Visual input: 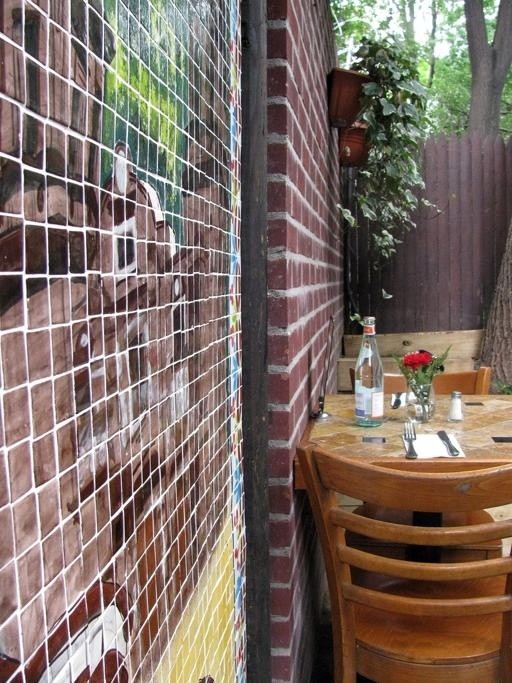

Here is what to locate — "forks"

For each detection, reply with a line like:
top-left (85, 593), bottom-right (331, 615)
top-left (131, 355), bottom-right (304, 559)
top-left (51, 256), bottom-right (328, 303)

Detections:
top-left (403, 421), bottom-right (418, 460)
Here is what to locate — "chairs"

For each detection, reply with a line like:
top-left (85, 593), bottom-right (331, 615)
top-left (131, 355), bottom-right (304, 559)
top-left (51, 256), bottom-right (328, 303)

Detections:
top-left (342, 366), bottom-right (504, 551)
top-left (295, 440), bottom-right (512, 683)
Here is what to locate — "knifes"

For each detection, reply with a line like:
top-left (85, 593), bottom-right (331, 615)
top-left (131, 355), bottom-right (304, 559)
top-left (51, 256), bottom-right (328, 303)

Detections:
top-left (437, 429), bottom-right (460, 457)
top-left (391, 390), bottom-right (402, 410)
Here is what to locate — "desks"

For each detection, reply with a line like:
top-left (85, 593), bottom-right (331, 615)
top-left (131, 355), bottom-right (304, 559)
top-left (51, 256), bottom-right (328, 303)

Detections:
top-left (296, 393), bottom-right (511, 564)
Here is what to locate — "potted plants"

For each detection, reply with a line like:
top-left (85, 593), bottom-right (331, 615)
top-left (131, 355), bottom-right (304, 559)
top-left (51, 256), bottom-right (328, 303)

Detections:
top-left (328, 31), bottom-right (441, 329)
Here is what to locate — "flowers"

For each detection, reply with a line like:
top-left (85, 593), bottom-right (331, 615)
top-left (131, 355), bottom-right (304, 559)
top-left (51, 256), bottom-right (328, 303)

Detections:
top-left (391, 345), bottom-right (456, 412)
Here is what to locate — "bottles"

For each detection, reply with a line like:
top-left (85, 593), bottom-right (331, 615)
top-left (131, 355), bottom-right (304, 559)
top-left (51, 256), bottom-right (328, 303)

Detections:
top-left (353, 312), bottom-right (385, 427)
top-left (448, 389), bottom-right (465, 423)
